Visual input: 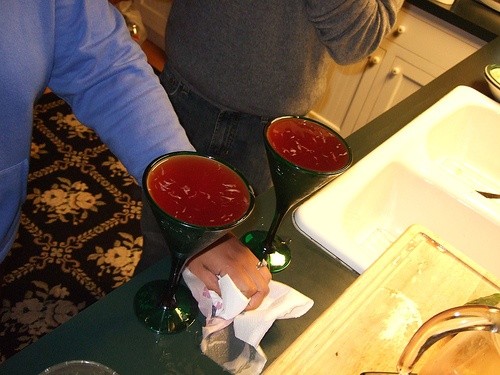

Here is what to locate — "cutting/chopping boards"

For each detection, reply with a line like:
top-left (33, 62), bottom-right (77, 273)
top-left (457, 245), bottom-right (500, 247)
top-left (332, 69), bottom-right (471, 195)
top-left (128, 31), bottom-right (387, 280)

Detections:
top-left (261, 223), bottom-right (500, 375)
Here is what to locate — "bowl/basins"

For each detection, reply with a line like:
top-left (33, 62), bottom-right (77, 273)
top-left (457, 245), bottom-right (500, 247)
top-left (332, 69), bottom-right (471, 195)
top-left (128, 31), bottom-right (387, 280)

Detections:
top-left (483, 63), bottom-right (500, 102)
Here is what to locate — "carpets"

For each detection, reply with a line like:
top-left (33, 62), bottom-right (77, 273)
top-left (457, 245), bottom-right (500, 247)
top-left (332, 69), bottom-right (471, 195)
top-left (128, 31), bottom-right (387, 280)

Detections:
top-left (0, 63), bottom-right (162, 361)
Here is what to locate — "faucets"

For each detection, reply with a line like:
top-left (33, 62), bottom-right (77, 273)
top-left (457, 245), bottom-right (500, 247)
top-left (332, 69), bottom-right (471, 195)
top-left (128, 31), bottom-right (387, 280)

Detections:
top-left (396, 306), bottom-right (500, 375)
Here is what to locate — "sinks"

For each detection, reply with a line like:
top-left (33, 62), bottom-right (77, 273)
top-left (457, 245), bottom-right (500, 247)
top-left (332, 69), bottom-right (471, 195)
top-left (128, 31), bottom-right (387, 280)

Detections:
top-left (335, 161), bottom-right (500, 273)
top-left (415, 101), bottom-right (499, 210)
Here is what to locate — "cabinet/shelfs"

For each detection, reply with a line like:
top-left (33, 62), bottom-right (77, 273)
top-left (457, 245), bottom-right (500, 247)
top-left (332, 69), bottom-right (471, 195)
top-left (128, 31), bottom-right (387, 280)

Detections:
top-left (306, 1), bottom-right (487, 136)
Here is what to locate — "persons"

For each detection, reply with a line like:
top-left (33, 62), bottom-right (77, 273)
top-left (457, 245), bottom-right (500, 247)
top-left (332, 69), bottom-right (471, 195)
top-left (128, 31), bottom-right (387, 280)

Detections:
top-left (131, 0), bottom-right (404, 278)
top-left (0, 0), bottom-right (272, 312)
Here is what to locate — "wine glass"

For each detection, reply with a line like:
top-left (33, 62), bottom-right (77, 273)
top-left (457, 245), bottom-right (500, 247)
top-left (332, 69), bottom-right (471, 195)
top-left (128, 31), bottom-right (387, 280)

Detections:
top-left (240, 114), bottom-right (353, 273)
top-left (134, 150), bottom-right (256, 335)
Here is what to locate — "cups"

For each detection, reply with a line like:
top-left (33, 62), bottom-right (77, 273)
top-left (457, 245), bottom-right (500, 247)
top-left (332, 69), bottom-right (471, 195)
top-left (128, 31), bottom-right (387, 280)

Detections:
top-left (398, 305), bottom-right (500, 375)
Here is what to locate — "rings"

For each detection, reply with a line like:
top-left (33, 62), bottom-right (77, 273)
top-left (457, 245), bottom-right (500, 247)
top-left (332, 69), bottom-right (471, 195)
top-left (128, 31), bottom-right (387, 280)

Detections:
top-left (256, 259), bottom-right (267, 270)
